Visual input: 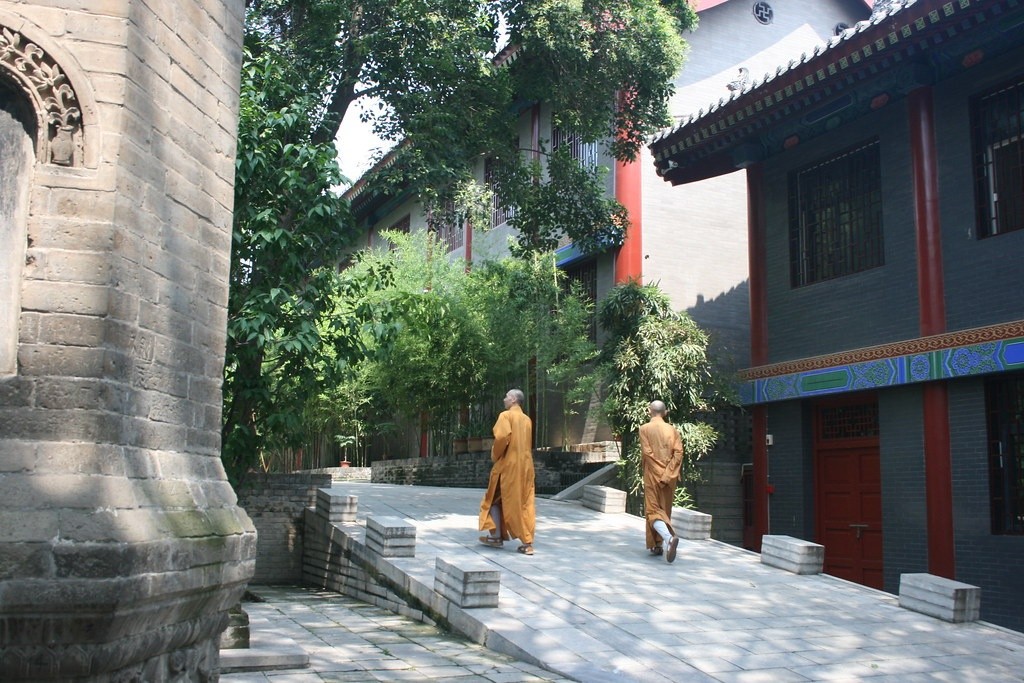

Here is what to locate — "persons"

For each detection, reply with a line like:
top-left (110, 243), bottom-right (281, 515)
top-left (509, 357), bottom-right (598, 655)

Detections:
top-left (478, 388), bottom-right (536, 556)
top-left (639, 399), bottom-right (684, 564)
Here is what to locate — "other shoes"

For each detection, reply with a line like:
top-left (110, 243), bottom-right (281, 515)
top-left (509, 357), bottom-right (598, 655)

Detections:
top-left (650, 546), bottom-right (664, 556)
top-left (478, 535), bottom-right (503, 546)
top-left (516, 544), bottom-right (534, 556)
top-left (666, 536), bottom-right (680, 565)
